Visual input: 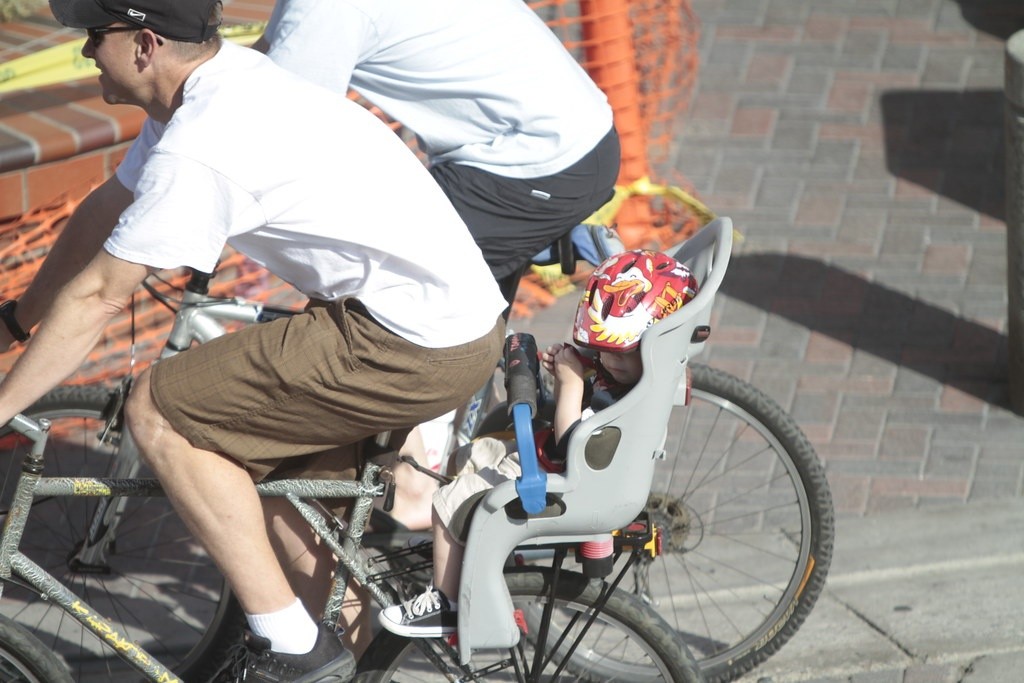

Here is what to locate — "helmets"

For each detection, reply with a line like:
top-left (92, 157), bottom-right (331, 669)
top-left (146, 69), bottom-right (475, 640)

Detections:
top-left (573, 249), bottom-right (701, 352)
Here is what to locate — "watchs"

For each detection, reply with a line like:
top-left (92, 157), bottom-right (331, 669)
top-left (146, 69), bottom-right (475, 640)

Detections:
top-left (0, 300), bottom-right (30, 342)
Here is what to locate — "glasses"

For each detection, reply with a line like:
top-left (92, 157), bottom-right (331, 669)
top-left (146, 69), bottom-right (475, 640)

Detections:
top-left (88, 25), bottom-right (164, 47)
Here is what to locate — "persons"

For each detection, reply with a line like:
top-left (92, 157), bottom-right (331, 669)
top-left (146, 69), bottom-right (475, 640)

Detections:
top-left (377, 249), bottom-right (699, 637)
top-left (250, 0), bottom-right (621, 549)
top-left (0, 0), bottom-right (509, 682)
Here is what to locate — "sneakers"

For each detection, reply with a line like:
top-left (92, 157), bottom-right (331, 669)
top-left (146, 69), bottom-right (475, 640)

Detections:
top-left (402, 536), bottom-right (436, 575)
top-left (207, 622), bottom-right (356, 683)
top-left (376, 578), bottom-right (458, 639)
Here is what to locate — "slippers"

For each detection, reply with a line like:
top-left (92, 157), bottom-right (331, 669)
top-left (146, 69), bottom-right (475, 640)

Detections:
top-left (330, 506), bottom-right (437, 571)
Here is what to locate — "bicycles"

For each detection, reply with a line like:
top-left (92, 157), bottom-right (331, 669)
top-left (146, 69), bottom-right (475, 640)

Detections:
top-left (0, 190), bottom-right (837, 683)
top-left (1, 415), bottom-right (705, 683)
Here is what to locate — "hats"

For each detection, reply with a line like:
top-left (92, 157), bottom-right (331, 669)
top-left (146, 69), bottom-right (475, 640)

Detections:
top-left (47, 0), bottom-right (223, 44)
